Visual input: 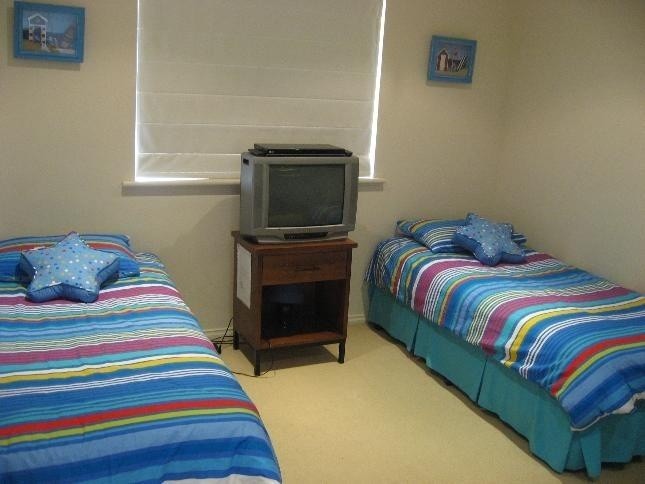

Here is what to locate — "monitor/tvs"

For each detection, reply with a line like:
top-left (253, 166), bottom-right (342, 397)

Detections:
top-left (239, 152), bottom-right (359, 245)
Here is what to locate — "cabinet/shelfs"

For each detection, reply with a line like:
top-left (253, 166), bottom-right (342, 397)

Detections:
top-left (229, 230), bottom-right (358, 376)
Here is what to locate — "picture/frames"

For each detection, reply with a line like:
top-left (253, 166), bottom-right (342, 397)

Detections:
top-left (13, 0), bottom-right (86, 63)
top-left (427, 34), bottom-right (477, 84)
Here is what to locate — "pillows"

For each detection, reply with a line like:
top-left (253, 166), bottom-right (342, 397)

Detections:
top-left (396, 212), bottom-right (527, 266)
top-left (0, 232), bottom-right (142, 304)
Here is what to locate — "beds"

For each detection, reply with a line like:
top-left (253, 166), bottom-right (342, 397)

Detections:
top-left (0, 233), bottom-right (281, 483)
top-left (369, 215), bottom-right (645, 480)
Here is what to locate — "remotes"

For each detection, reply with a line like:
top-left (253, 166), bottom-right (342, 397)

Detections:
top-left (248, 148), bottom-right (266, 157)
